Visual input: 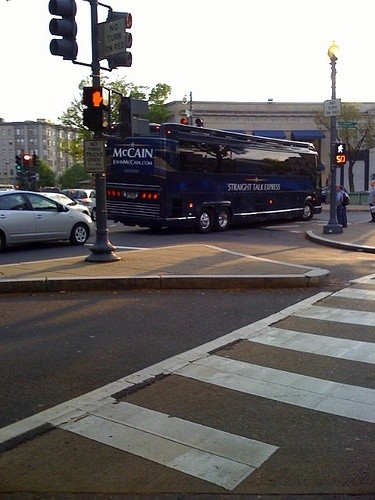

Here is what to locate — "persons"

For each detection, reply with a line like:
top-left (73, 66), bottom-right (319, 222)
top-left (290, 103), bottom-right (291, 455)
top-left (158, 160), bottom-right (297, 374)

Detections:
top-left (70, 191), bottom-right (96, 199)
top-left (336, 185), bottom-right (349, 228)
top-left (369, 179), bottom-right (375, 222)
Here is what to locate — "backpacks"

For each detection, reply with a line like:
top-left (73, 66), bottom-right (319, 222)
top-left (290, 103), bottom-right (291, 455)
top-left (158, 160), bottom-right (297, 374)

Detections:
top-left (339, 191), bottom-right (350, 206)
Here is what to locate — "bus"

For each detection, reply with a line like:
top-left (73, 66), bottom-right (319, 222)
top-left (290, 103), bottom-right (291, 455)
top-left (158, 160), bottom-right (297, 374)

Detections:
top-left (95, 122), bottom-right (325, 234)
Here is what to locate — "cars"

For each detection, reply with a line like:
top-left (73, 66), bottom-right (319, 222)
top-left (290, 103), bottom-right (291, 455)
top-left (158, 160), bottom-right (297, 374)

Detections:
top-left (0, 190), bottom-right (97, 254)
top-left (0, 184), bottom-right (96, 221)
top-left (321, 184), bottom-right (350, 207)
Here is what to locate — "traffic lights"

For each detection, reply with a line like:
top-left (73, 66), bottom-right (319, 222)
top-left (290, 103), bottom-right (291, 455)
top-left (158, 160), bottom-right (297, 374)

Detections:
top-left (100, 86), bottom-right (110, 111)
top-left (88, 87), bottom-right (102, 109)
top-left (15, 154), bottom-right (23, 170)
top-left (334, 141), bottom-right (347, 166)
top-left (33, 154), bottom-right (40, 166)
top-left (48, 0), bottom-right (78, 60)
top-left (106, 8), bottom-right (132, 69)
top-left (94, 108), bottom-right (111, 131)
top-left (180, 117), bottom-right (189, 125)
top-left (22, 153), bottom-right (30, 163)
top-left (195, 118), bottom-right (204, 128)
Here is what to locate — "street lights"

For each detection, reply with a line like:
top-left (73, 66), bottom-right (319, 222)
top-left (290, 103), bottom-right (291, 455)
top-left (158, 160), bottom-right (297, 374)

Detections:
top-left (322, 40), bottom-right (344, 235)
top-left (182, 90), bottom-right (193, 126)
